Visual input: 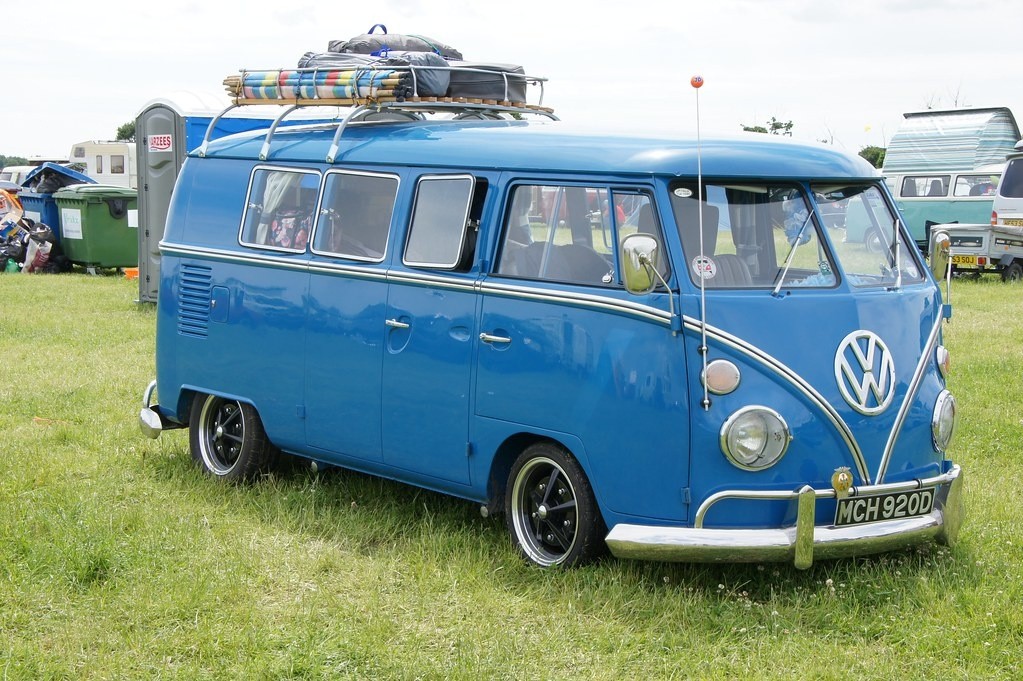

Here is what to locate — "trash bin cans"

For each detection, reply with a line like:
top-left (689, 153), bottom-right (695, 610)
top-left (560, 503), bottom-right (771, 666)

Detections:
top-left (52, 185), bottom-right (138, 276)
top-left (17, 162), bottom-right (99, 247)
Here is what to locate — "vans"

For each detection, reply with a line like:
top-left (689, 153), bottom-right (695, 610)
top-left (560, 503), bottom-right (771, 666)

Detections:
top-left (1, 166), bottom-right (41, 192)
top-left (135, 63), bottom-right (965, 576)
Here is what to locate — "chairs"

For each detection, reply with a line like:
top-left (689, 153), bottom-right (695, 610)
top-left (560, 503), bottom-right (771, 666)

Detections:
top-left (525, 240), bottom-right (613, 283)
top-left (927, 180), bottom-right (942, 196)
top-left (903, 179), bottom-right (917, 197)
top-left (693, 254), bottom-right (755, 287)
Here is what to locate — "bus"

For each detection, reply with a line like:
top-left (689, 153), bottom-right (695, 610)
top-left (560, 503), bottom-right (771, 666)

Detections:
top-left (844, 164), bottom-right (1006, 257)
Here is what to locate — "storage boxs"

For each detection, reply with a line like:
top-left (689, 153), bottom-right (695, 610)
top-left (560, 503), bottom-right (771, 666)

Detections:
top-left (0, 207), bottom-right (31, 243)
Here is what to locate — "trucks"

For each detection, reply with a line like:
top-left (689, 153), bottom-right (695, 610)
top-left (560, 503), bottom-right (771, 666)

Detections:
top-left (929, 153), bottom-right (1022, 283)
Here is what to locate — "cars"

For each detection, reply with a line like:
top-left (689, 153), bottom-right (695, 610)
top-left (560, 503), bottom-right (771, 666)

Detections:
top-left (815, 195), bottom-right (846, 226)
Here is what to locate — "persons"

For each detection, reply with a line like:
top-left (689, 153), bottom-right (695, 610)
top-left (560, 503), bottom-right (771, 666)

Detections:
top-left (602, 199), bottom-right (626, 227)
